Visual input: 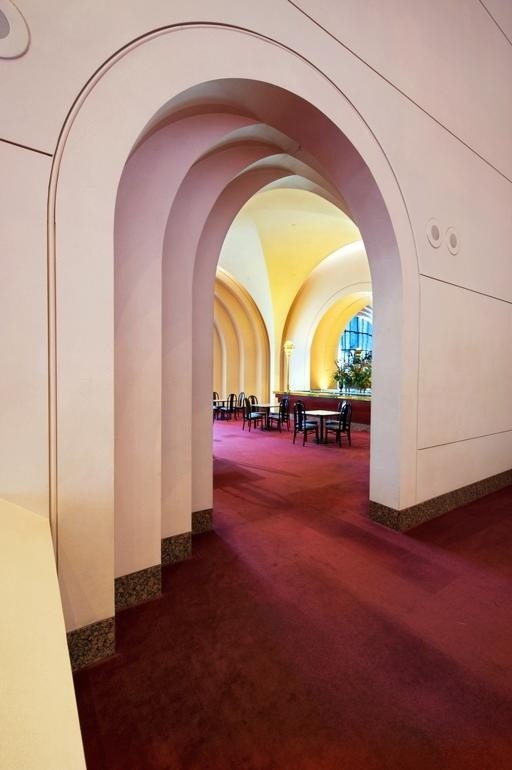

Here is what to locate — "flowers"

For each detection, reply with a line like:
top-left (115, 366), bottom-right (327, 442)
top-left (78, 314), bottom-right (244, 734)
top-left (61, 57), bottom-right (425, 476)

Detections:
top-left (332, 350), bottom-right (372, 390)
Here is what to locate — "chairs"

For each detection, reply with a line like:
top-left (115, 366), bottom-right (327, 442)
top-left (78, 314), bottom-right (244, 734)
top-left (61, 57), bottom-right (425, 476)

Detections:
top-left (212, 392), bottom-right (354, 449)
